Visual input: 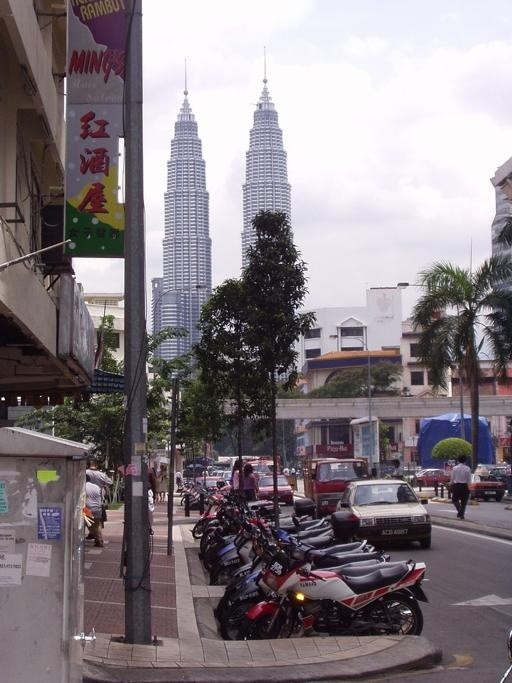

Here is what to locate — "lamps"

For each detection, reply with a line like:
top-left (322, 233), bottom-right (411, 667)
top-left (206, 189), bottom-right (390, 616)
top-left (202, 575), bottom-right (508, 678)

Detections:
top-left (0, 238), bottom-right (76, 272)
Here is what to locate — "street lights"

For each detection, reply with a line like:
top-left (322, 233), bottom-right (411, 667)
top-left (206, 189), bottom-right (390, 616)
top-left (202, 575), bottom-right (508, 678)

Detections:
top-left (397, 283), bottom-right (465, 441)
top-left (154, 286), bottom-right (206, 380)
top-left (329, 334), bottom-right (373, 480)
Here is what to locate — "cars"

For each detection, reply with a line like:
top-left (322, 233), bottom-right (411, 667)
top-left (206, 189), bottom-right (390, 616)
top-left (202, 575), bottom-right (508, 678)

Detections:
top-left (466, 467), bottom-right (512, 503)
top-left (333, 480), bottom-right (431, 551)
top-left (195, 459), bottom-right (280, 492)
top-left (256, 475), bottom-right (294, 506)
top-left (414, 468), bottom-right (450, 486)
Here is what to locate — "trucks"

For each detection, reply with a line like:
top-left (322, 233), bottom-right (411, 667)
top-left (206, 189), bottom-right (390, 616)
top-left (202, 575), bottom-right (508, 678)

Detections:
top-left (300, 456), bottom-right (370, 519)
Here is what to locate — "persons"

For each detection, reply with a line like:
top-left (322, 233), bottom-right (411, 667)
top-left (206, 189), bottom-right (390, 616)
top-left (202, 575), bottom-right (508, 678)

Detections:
top-left (244, 463), bottom-right (259, 501)
top-left (231, 459), bottom-right (240, 496)
top-left (86, 458), bottom-right (113, 487)
top-left (176, 470), bottom-right (182, 489)
top-left (449, 454), bottom-right (471, 520)
top-left (86, 474), bottom-right (104, 547)
top-left (147, 464), bottom-right (167, 534)
top-left (392, 459), bottom-right (403, 480)
top-left (371, 463), bottom-right (378, 479)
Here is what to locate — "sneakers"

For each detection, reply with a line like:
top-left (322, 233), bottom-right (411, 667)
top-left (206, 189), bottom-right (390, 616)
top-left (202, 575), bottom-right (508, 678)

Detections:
top-left (86, 534), bottom-right (103, 547)
top-left (457, 513), bottom-right (464, 519)
